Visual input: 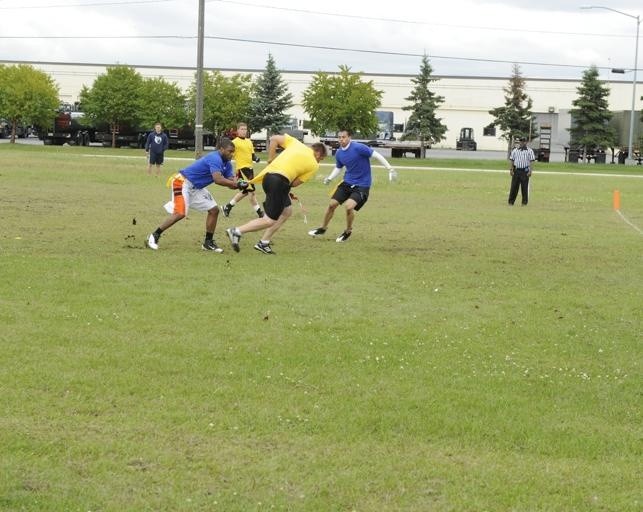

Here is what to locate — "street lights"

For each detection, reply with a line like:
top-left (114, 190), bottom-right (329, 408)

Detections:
top-left (577, 3), bottom-right (643, 166)
top-left (612, 66), bottom-right (643, 76)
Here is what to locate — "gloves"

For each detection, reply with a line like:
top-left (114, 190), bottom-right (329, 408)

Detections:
top-left (236, 179), bottom-right (249, 191)
top-left (322, 177), bottom-right (332, 185)
top-left (387, 168), bottom-right (398, 183)
top-left (255, 156), bottom-right (261, 163)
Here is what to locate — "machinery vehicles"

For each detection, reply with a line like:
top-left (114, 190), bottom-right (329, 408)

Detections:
top-left (0, 101), bottom-right (212, 149)
top-left (456, 126), bottom-right (479, 151)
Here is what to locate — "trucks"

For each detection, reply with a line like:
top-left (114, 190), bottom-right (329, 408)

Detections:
top-left (246, 111), bottom-right (300, 154)
top-left (511, 104), bottom-right (643, 167)
top-left (369, 108), bottom-right (394, 146)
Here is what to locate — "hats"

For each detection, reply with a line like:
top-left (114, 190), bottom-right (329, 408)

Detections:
top-left (518, 136), bottom-right (528, 142)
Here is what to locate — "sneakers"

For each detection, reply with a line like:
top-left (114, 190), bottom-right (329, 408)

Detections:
top-left (200, 238), bottom-right (225, 254)
top-left (220, 201), bottom-right (232, 218)
top-left (224, 226), bottom-right (242, 253)
top-left (334, 229), bottom-right (352, 243)
top-left (145, 231), bottom-right (160, 250)
top-left (305, 225), bottom-right (327, 239)
top-left (253, 239), bottom-right (277, 255)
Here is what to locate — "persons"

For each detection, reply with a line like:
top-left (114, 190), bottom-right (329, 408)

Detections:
top-left (145, 123), bottom-right (169, 176)
top-left (509, 137), bottom-right (535, 205)
top-left (84, 131), bottom-right (90, 146)
top-left (144, 132), bottom-right (148, 148)
top-left (307, 127), bottom-right (397, 243)
top-left (138, 132), bottom-right (143, 148)
top-left (220, 123), bottom-right (264, 218)
top-left (78, 131), bottom-right (83, 146)
top-left (145, 139), bottom-right (249, 253)
top-left (225, 134), bottom-right (328, 254)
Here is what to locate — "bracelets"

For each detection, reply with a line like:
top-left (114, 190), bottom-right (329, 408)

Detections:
top-left (510, 169), bottom-right (514, 171)
